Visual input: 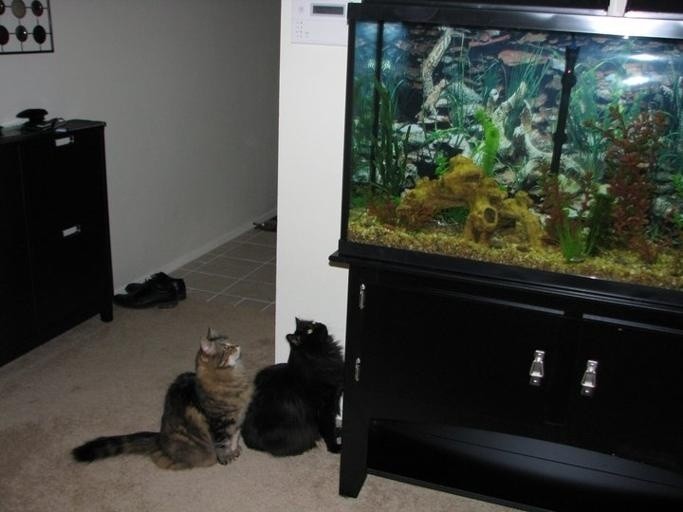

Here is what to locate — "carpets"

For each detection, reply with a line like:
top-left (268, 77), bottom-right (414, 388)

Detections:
top-left (0, 296), bottom-right (532, 511)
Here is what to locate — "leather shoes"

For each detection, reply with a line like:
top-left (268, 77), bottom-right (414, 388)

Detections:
top-left (113, 277), bottom-right (177, 308)
top-left (125, 271), bottom-right (186, 301)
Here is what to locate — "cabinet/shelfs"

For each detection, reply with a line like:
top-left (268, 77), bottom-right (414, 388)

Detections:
top-left (328, 249), bottom-right (683, 511)
top-left (0, 119), bottom-right (114, 368)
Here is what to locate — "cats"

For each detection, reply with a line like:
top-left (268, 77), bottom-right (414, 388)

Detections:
top-left (241, 315), bottom-right (347, 458)
top-left (69, 328), bottom-right (246, 470)
